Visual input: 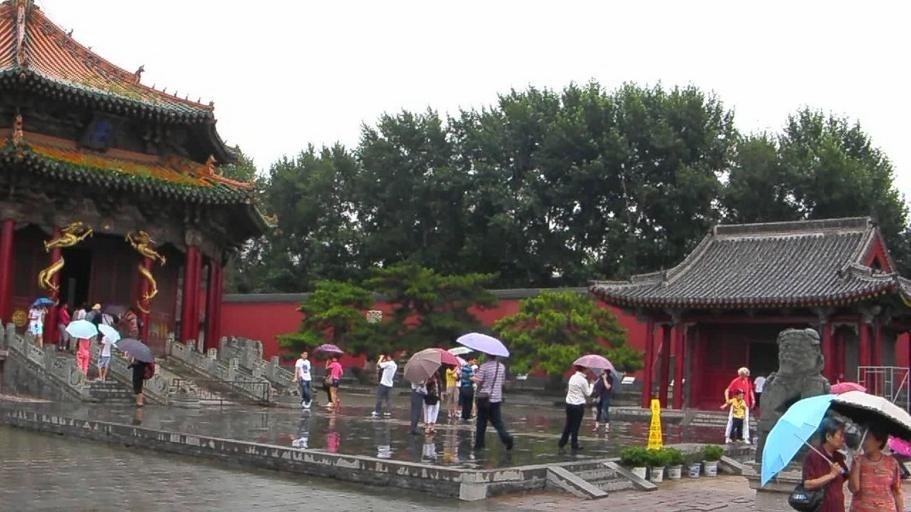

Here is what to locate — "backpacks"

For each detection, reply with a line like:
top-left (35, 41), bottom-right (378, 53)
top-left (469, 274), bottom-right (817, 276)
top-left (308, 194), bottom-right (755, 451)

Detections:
top-left (144, 361), bottom-right (154, 380)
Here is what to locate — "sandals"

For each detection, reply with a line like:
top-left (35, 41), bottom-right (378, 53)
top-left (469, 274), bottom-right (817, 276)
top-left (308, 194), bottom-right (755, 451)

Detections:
top-left (592, 426), bottom-right (610, 433)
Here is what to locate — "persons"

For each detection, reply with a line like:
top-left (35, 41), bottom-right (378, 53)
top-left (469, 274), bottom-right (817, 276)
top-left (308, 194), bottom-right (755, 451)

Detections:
top-left (293, 350), bottom-right (315, 410)
top-left (371, 351), bottom-right (399, 415)
top-left (800, 416), bottom-right (852, 512)
top-left (13, 302), bottom-right (139, 382)
top-left (847, 424), bottom-right (905, 511)
top-left (721, 389), bottom-right (748, 441)
top-left (326, 355), bottom-right (346, 408)
top-left (752, 370), bottom-right (767, 406)
top-left (125, 357), bottom-right (147, 407)
top-left (591, 369), bottom-right (614, 433)
top-left (472, 354), bottom-right (516, 450)
top-left (555, 366), bottom-right (595, 450)
top-left (404, 356), bottom-right (479, 437)
top-left (725, 366), bottom-right (757, 444)
top-left (377, 352), bottom-right (387, 383)
top-left (323, 356), bottom-right (334, 407)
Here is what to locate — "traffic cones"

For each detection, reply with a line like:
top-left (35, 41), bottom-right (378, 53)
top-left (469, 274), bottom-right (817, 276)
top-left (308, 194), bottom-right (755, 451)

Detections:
top-left (647, 398), bottom-right (667, 452)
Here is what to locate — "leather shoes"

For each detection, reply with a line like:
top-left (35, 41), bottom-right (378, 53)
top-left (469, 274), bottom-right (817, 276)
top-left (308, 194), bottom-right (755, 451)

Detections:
top-left (472, 441), bottom-right (486, 451)
top-left (557, 444), bottom-right (583, 450)
top-left (504, 435), bottom-right (514, 451)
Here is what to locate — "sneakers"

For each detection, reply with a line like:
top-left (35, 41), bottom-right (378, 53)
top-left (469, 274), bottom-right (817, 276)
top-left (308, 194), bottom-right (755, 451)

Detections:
top-left (408, 427), bottom-right (438, 435)
top-left (300, 399), bottom-right (313, 409)
top-left (371, 411), bottom-right (392, 417)
top-left (447, 409), bottom-right (476, 421)
top-left (724, 436), bottom-right (752, 446)
top-left (324, 400), bottom-right (341, 410)
top-left (94, 377), bottom-right (106, 384)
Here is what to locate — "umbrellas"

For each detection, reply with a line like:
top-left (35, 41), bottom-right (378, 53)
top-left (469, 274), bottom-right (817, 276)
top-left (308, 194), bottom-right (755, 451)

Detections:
top-left (572, 353), bottom-right (619, 372)
top-left (447, 345), bottom-right (475, 356)
top-left (593, 368), bottom-right (622, 391)
top-left (829, 390), bottom-right (911, 457)
top-left (312, 343), bottom-right (346, 356)
top-left (31, 298), bottom-right (55, 312)
top-left (403, 347), bottom-right (441, 383)
top-left (830, 379), bottom-right (869, 394)
top-left (456, 331), bottom-right (512, 360)
top-left (115, 338), bottom-right (155, 364)
top-left (433, 347), bottom-right (462, 366)
top-left (759, 394), bottom-right (837, 486)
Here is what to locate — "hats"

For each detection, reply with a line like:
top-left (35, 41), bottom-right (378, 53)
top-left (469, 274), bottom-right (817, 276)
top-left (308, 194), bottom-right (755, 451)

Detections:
top-left (91, 302), bottom-right (102, 310)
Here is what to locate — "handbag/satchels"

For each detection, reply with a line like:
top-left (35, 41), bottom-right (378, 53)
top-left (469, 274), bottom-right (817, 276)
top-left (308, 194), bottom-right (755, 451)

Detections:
top-left (425, 394), bottom-right (437, 405)
top-left (788, 483), bottom-right (825, 512)
top-left (474, 392), bottom-right (490, 404)
top-left (324, 376), bottom-right (333, 384)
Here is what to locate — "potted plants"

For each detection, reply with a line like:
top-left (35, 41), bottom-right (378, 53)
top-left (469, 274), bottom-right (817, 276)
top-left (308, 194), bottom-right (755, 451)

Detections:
top-left (626, 442), bottom-right (648, 484)
top-left (685, 455), bottom-right (704, 481)
top-left (704, 444), bottom-right (725, 479)
top-left (665, 449), bottom-right (684, 481)
top-left (643, 444), bottom-right (667, 485)
